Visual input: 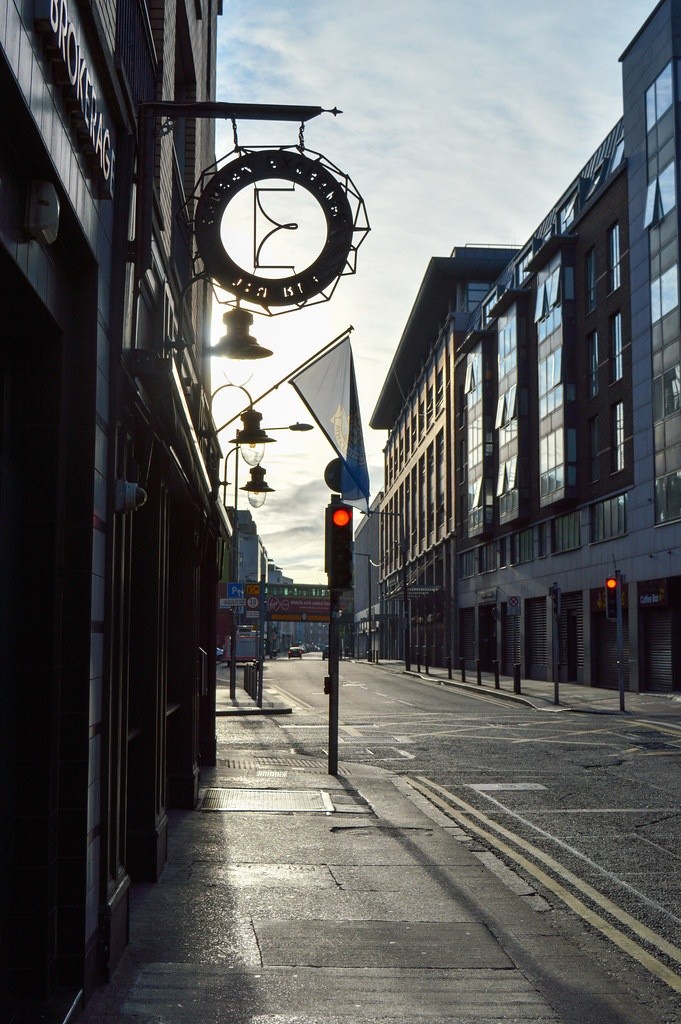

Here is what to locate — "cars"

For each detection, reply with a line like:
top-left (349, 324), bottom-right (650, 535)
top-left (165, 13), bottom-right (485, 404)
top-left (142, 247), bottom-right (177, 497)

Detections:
top-left (321, 645), bottom-right (331, 660)
top-left (287, 647), bottom-right (302, 659)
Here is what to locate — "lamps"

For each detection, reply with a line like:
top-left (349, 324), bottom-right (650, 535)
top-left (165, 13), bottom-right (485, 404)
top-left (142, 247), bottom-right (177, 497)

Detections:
top-left (22, 175), bottom-right (62, 244)
top-left (213, 446), bottom-right (277, 508)
top-left (199, 383), bottom-right (278, 465)
top-left (165, 273), bottom-right (275, 387)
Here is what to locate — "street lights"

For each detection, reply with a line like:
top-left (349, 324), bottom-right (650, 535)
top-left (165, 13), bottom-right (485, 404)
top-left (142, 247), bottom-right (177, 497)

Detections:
top-left (229, 423), bottom-right (314, 700)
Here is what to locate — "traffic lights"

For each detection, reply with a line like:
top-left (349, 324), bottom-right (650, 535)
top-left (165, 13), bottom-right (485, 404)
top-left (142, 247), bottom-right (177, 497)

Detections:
top-left (605, 576), bottom-right (619, 621)
top-left (329, 503), bottom-right (353, 592)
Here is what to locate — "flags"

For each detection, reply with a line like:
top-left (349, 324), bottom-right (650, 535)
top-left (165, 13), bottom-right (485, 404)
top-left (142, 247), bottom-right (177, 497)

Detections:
top-left (289, 336), bottom-right (372, 519)
